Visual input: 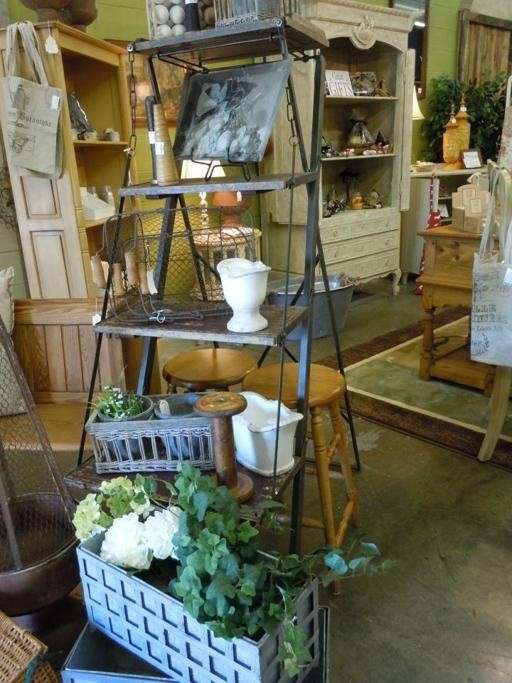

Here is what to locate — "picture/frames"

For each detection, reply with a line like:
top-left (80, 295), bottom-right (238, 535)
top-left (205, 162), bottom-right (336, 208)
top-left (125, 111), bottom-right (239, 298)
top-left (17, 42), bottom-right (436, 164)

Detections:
top-left (101, 37), bottom-right (190, 128)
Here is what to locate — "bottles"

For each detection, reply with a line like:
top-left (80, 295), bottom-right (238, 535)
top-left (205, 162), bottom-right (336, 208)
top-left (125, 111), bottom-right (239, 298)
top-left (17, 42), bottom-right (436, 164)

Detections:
top-left (326, 184), bottom-right (339, 214)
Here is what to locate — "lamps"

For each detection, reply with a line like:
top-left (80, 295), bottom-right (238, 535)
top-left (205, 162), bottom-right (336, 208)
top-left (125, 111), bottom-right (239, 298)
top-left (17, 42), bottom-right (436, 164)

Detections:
top-left (178, 151), bottom-right (227, 242)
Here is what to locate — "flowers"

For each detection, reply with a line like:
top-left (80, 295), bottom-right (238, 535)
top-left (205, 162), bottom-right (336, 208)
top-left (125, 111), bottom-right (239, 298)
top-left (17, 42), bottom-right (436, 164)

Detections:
top-left (89, 384), bottom-right (144, 419)
top-left (71, 473), bottom-right (394, 631)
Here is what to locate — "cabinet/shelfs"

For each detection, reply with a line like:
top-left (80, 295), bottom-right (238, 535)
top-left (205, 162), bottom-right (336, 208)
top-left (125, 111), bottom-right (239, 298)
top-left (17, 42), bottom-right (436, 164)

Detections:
top-left (256, 0), bottom-right (425, 302)
top-left (0, 18), bottom-right (161, 406)
top-left (400, 160), bottom-right (488, 284)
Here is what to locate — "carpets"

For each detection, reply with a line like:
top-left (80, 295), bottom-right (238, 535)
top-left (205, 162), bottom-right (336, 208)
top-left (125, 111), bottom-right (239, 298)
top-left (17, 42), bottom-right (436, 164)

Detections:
top-left (313, 304), bottom-right (511, 472)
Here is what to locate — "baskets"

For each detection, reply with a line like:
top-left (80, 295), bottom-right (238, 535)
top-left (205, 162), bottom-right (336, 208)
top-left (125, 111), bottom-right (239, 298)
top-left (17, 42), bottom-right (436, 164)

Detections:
top-left (0, 611), bottom-right (62, 683)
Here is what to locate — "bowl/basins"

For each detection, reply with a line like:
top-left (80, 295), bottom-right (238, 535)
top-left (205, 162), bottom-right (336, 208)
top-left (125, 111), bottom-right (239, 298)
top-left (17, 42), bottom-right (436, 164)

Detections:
top-left (252, 276), bottom-right (355, 337)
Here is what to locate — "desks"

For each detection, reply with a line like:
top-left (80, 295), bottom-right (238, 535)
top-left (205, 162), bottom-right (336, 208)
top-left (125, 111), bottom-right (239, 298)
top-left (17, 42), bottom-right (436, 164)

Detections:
top-left (192, 227), bottom-right (262, 302)
top-left (412, 226), bottom-right (510, 397)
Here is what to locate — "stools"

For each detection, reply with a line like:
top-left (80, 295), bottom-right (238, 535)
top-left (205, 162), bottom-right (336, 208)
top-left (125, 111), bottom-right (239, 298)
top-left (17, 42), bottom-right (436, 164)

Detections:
top-left (238, 361), bottom-right (359, 555)
top-left (163, 347), bottom-right (259, 396)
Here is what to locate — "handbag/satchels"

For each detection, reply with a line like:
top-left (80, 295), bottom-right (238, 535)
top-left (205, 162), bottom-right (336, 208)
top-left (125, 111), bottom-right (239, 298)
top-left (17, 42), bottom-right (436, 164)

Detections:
top-left (1, 20), bottom-right (66, 181)
top-left (465, 165), bottom-right (512, 368)
